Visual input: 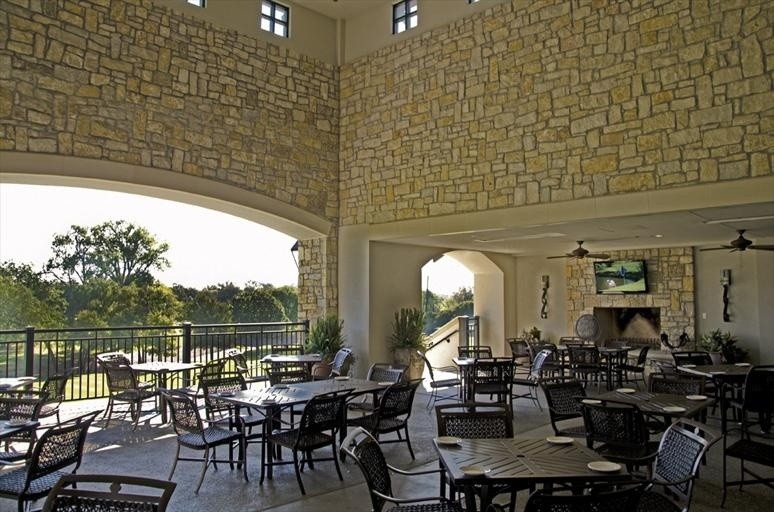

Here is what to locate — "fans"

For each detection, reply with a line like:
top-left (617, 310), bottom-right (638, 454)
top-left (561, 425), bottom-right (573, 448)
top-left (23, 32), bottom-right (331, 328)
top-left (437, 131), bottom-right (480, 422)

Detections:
top-left (546, 240), bottom-right (611, 260)
top-left (699, 229), bottom-right (774, 253)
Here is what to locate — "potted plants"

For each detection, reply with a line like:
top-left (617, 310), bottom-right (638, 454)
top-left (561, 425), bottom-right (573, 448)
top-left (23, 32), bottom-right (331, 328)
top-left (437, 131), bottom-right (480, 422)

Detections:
top-left (385, 306), bottom-right (434, 384)
top-left (697, 330), bottom-right (738, 365)
top-left (303, 316), bottom-right (351, 376)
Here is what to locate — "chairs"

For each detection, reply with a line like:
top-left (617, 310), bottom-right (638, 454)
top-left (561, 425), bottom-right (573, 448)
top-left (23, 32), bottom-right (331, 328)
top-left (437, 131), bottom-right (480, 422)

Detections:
top-left (197, 371), bottom-right (277, 469)
top-left (559, 337), bottom-right (585, 346)
top-left (648, 372), bottom-right (706, 396)
top-left (507, 338), bottom-right (529, 374)
top-left (457, 346), bottom-right (492, 358)
top-left (340, 426), bottom-right (462, 512)
top-left (30, 474), bottom-right (178, 512)
top-left (417, 350), bottom-right (465, 415)
top-left (600, 417), bottom-right (722, 512)
top-left (156, 386), bottom-right (249, 493)
top-left (271, 344), bottom-right (304, 355)
top-left (97, 357), bottom-right (161, 432)
top-left (432, 438), bottom-right (632, 512)
top-left (345, 377), bottom-right (425, 465)
top-left (528, 341), bottom-right (570, 383)
top-left (565, 343), bottom-right (608, 395)
top-left (726, 365), bottom-right (774, 440)
top-left (540, 381), bottom-right (587, 438)
top-left (614, 345), bottom-right (651, 390)
top-left (310, 348), bottom-right (351, 381)
top-left (468, 356), bottom-right (515, 419)
top-left (671, 351), bottom-right (713, 366)
top-left (0, 390), bottom-right (50, 468)
top-left (524, 480), bottom-right (648, 512)
top-left (602, 340), bottom-right (628, 384)
top-left (228, 352), bottom-right (270, 388)
top-left (650, 359), bottom-right (678, 371)
top-left (264, 363), bottom-right (312, 386)
top-left (97, 352), bottom-right (155, 418)
top-left (0, 409), bottom-right (105, 512)
top-left (435, 402), bottom-right (536, 512)
top-left (259, 388), bottom-right (356, 495)
top-left (334, 363), bottom-right (409, 440)
top-left (504, 349), bottom-right (552, 411)
top-left (570, 395), bottom-right (661, 480)
top-left (0, 370), bottom-right (74, 431)
top-left (168, 356), bottom-right (230, 424)
top-left (721, 417), bottom-right (774, 509)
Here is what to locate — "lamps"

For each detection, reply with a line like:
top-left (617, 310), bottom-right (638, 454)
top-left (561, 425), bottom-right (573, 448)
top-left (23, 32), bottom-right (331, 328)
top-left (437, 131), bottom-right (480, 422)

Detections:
top-left (541, 275), bottom-right (549, 321)
top-left (720, 268), bottom-right (732, 322)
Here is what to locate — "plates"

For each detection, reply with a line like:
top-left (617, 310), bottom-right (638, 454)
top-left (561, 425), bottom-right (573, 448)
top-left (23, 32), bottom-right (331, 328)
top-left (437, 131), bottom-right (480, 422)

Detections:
top-left (334, 376), bottom-right (350, 380)
top-left (546, 437), bottom-right (574, 444)
top-left (709, 372), bottom-right (726, 375)
top-left (607, 349), bottom-right (617, 351)
top-left (262, 399), bottom-right (276, 404)
top-left (581, 399), bottom-right (601, 404)
top-left (621, 347), bottom-right (631, 349)
top-left (273, 384), bottom-right (287, 388)
top-left (3, 421), bottom-right (32, 427)
top-left (217, 393), bottom-right (235, 396)
top-left (662, 407), bottom-right (686, 413)
top-left (735, 363), bottom-right (750, 366)
top-left (433, 437), bottom-right (461, 444)
top-left (377, 382), bottom-right (395, 386)
top-left (265, 359), bottom-right (271, 361)
top-left (685, 395), bottom-right (707, 400)
top-left (616, 388), bottom-right (635, 393)
top-left (682, 365), bottom-right (696, 368)
top-left (458, 466), bottom-right (490, 476)
top-left (587, 462), bottom-right (621, 472)
top-left (271, 354), bottom-right (278, 356)
